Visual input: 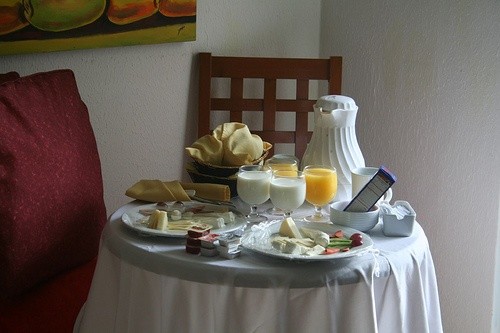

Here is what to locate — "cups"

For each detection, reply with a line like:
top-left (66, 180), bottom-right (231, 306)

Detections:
top-left (271, 154), bottom-right (299, 165)
top-left (351, 167), bottom-right (393, 207)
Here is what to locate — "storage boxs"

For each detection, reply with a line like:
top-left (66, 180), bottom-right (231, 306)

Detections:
top-left (381, 197), bottom-right (418, 238)
top-left (343, 164), bottom-right (399, 212)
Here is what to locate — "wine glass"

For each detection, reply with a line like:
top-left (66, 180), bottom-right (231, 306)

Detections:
top-left (269, 169), bottom-right (307, 221)
top-left (263, 159), bottom-right (299, 215)
top-left (236, 165), bottom-right (272, 223)
top-left (303, 165), bottom-right (337, 222)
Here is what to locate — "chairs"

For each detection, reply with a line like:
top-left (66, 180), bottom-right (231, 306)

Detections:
top-left (0, 70), bottom-right (97, 333)
top-left (196, 47), bottom-right (344, 168)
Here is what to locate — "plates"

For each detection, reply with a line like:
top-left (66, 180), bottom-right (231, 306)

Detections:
top-left (122, 202), bottom-right (247, 237)
top-left (241, 221), bottom-right (374, 262)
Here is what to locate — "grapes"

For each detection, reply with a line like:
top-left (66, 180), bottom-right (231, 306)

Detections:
top-left (350, 234), bottom-right (364, 245)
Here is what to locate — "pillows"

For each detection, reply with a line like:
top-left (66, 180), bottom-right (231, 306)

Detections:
top-left (0, 68), bottom-right (109, 302)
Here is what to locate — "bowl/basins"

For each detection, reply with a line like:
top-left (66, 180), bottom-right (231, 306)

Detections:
top-left (185, 151), bottom-right (269, 196)
top-left (329, 202), bottom-right (380, 233)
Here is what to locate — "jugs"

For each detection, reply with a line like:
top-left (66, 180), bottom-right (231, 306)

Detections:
top-left (301, 94), bottom-right (365, 203)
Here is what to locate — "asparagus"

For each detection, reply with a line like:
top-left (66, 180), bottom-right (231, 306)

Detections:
top-left (327, 239), bottom-right (352, 248)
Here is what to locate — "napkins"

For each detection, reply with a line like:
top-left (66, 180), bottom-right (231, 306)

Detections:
top-left (125, 174), bottom-right (232, 207)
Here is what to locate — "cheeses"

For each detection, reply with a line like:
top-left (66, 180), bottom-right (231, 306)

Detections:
top-left (148, 210), bottom-right (167, 231)
top-left (271, 217), bottom-right (331, 256)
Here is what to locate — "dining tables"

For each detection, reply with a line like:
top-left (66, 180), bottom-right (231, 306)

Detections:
top-left (72, 196), bottom-right (445, 333)
